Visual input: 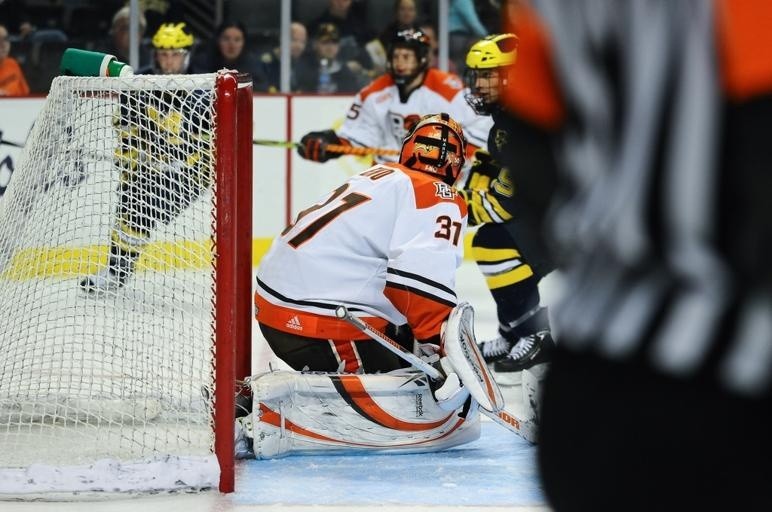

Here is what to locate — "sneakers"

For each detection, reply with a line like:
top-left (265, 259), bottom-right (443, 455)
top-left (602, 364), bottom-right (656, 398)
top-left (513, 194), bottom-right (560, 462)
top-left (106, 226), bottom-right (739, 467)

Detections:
top-left (477, 338), bottom-right (520, 364)
top-left (496, 331), bottom-right (554, 372)
top-left (80, 274), bottom-right (126, 293)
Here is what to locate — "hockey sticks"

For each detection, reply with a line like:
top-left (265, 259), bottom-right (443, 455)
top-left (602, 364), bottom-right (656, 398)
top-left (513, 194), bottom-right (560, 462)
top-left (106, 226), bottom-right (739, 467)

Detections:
top-left (334, 303), bottom-right (540, 445)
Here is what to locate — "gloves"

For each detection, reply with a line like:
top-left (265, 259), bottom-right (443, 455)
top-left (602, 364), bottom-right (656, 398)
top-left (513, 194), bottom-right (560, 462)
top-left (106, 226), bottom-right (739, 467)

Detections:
top-left (298, 132), bottom-right (343, 162)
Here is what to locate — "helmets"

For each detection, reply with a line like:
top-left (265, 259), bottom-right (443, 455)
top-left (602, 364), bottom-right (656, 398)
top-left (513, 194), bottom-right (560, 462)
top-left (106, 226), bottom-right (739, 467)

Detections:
top-left (386, 26), bottom-right (431, 86)
top-left (399, 114), bottom-right (467, 186)
top-left (152, 21), bottom-right (194, 49)
top-left (464, 32), bottom-right (519, 115)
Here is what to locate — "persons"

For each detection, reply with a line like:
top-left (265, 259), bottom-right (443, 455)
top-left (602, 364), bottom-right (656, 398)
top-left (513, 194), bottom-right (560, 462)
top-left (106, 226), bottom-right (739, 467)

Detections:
top-left (0, 0), bottom-right (437, 98)
top-left (235, 30), bottom-right (554, 457)
top-left (82, 21), bottom-right (212, 296)
